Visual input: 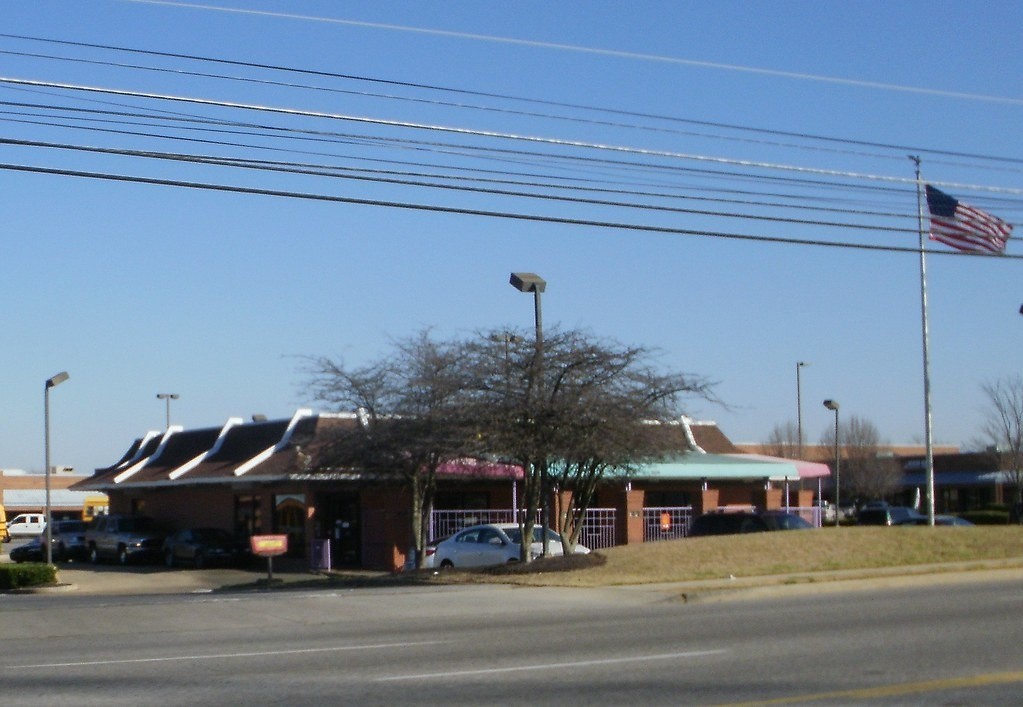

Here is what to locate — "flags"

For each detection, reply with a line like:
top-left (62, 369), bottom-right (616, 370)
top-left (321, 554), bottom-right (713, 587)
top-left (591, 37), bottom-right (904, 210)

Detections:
top-left (924, 184), bottom-right (1013, 255)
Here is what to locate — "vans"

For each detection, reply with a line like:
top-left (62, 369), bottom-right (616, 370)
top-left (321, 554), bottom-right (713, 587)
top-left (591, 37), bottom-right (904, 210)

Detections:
top-left (82, 495), bottom-right (109, 522)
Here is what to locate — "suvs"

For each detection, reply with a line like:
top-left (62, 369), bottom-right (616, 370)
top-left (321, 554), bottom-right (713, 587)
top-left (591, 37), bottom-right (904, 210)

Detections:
top-left (39, 520), bottom-right (93, 561)
top-left (857, 503), bottom-right (920, 526)
top-left (85, 512), bottom-right (165, 566)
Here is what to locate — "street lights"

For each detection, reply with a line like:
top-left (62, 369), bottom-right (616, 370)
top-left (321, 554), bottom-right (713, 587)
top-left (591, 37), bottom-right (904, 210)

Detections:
top-left (509, 271), bottom-right (547, 558)
top-left (796, 361), bottom-right (809, 490)
top-left (157, 393), bottom-right (180, 428)
top-left (44, 370), bottom-right (69, 563)
top-left (823, 399), bottom-right (839, 526)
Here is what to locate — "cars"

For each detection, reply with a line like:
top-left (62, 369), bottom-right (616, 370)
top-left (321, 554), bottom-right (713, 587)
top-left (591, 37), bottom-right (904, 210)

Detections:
top-left (434, 522), bottom-right (590, 568)
top-left (687, 511), bottom-right (814, 539)
top-left (162, 527), bottom-right (258, 571)
top-left (406, 535), bottom-right (447, 569)
top-left (4, 513), bottom-right (47, 539)
top-left (817, 501), bottom-right (834, 519)
top-left (9, 538), bottom-right (41, 564)
top-left (900, 516), bottom-right (976, 526)
